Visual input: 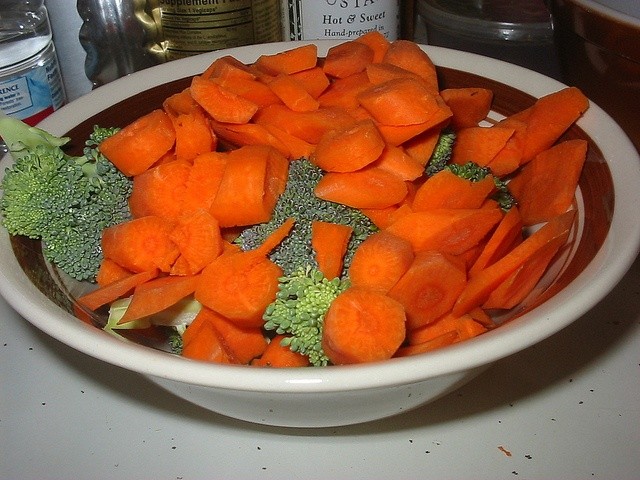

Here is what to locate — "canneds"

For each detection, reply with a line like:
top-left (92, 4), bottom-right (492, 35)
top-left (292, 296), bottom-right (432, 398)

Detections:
top-left (157, 2), bottom-right (282, 61)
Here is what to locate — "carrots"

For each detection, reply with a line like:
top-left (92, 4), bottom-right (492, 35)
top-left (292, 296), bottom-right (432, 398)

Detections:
top-left (6, 32), bottom-right (590, 367)
top-left (282, 0), bottom-right (399, 43)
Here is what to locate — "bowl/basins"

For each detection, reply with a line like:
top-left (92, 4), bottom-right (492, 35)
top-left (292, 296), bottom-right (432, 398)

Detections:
top-left (1, 36), bottom-right (639, 430)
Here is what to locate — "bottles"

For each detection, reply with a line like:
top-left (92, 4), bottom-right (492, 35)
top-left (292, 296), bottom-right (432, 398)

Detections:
top-left (1, 1), bottom-right (68, 161)
